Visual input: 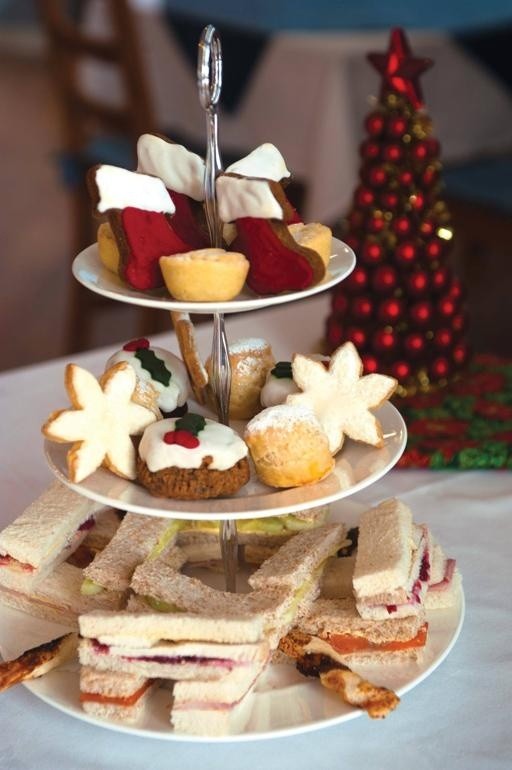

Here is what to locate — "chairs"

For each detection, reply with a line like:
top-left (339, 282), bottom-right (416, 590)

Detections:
top-left (36, 0), bottom-right (309, 355)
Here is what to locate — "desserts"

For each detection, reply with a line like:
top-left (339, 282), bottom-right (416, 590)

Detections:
top-left (1, 474), bottom-right (463, 736)
top-left (87, 131), bottom-right (333, 304)
top-left (43, 311), bottom-right (397, 499)
